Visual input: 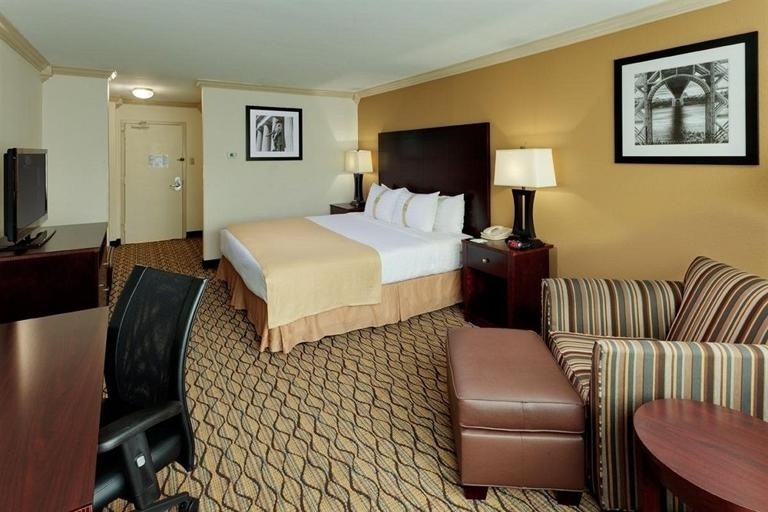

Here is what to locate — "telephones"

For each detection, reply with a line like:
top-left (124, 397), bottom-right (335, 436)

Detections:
top-left (480, 225), bottom-right (513, 241)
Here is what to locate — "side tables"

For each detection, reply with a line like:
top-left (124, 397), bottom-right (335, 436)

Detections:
top-left (631, 399), bottom-right (768, 512)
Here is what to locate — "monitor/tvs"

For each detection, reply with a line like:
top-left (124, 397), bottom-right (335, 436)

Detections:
top-left (3, 149), bottom-right (57, 249)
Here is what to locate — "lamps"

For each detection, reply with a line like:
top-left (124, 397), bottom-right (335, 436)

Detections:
top-left (131, 87), bottom-right (153, 100)
top-left (494, 146), bottom-right (557, 250)
top-left (343, 150), bottom-right (374, 208)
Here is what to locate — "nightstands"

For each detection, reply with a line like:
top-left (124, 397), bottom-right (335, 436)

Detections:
top-left (461, 237), bottom-right (554, 335)
top-left (329, 202), bottom-right (366, 214)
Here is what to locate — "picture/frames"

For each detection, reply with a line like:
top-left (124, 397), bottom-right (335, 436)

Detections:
top-left (246, 105), bottom-right (304, 161)
top-left (614, 29), bottom-right (760, 167)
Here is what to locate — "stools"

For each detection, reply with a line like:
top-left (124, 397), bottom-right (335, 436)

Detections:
top-left (446, 328), bottom-right (590, 506)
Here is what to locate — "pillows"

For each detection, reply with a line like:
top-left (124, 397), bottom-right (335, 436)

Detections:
top-left (363, 183), bottom-right (466, 245)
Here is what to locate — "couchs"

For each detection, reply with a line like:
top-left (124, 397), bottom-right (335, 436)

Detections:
top-left (541, 255), bottom-right (768, 512)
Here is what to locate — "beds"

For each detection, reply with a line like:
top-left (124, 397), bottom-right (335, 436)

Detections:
top-left (211, 122), bottom-right (490, 355)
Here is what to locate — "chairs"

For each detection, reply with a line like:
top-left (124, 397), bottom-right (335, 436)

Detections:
top-left (91, 264), bottom-right (208, 512)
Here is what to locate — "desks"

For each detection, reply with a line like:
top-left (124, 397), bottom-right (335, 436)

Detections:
top-left (0, 306), bottom-right (110, 512)
top-left (0, 222), bottom-right (111, 321)
